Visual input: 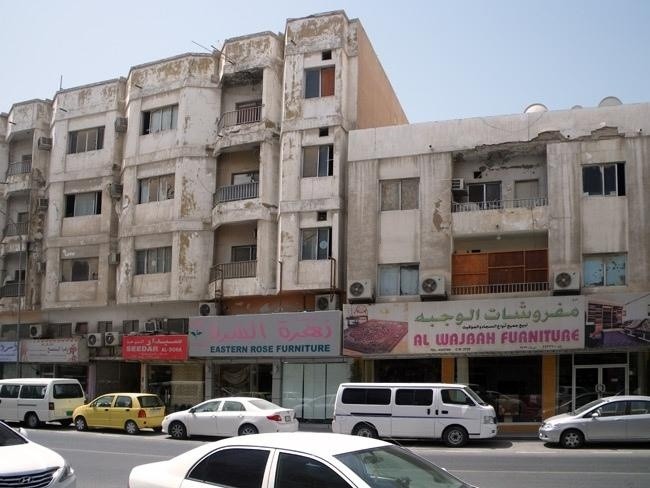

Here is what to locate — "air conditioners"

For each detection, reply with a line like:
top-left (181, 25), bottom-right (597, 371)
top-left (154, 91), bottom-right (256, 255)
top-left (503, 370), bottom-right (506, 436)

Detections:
top-left (552, 269), bottom-right (581, 291)
top-left (110, 252), bottom-right (120, 264)
top-left (37, 136), bottom-right (52, 150)
top-left (418, 275), bottom-right (446, 298)
top-left (453, 177), bottom-right (465, 189)
top-left (111, 183), bottom-right (122, 198)
top-left (29, 324), bottom-right (46, 338)
top-left (314, 279), bottom-right (376, 311)
top-left (86, 331), bottom-right (120, 348)
top-left (198, 302), bottom-right (220, 316)
top-left (115, 117), bottom-right (128, 133)
top-left (36, 197), bottom-right (49, 210)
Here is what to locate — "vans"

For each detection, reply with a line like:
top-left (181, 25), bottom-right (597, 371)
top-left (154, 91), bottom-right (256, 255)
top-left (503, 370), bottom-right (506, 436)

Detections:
top-left (0, 377), bottom-right (85, 427)
top-left (332, 382), bottom-right (499, 448)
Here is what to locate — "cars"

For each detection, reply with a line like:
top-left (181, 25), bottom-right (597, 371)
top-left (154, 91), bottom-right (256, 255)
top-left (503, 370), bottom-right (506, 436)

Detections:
top-left (496, 392), bottom-right (524, 414)
top-left (161, 396), bottom-right (299, 440)
top-left (538, 395), bottom-right (650, 449)
top-left (293, 393), bottom-right (337, 419)
top-left (128, 429), bottom-right (479, 487)
top-left (0, 419), bottom-right (78, 488)
top-left (72, 392), bottom-right (166, 435)
top-left (542, 389), bottom-right (611, 416)
